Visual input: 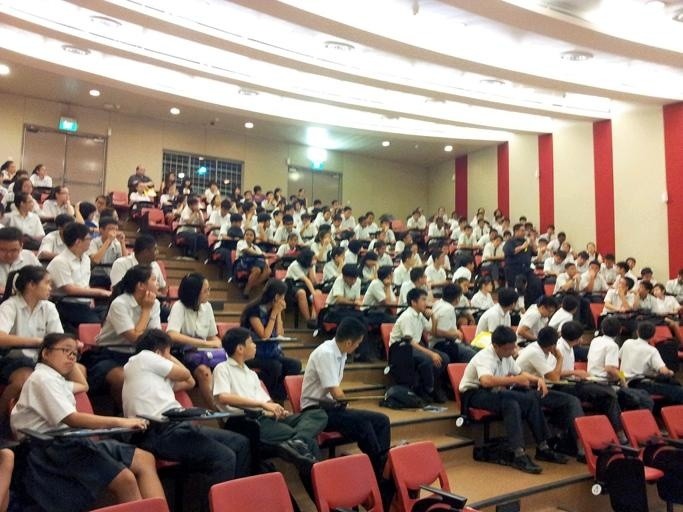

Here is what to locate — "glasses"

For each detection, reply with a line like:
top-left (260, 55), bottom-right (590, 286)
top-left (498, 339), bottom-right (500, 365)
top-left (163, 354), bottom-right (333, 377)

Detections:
top-left (54, 348), bottom-right (82, 362)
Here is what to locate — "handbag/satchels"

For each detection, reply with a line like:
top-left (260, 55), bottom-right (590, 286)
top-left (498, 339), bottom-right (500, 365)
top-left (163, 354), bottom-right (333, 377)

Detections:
top-left (183, 345), bottom-right (227, 369)
top-left (380, 386), bottom-right (425, 409)
top-left (474, 436), bottom-right (512, 466)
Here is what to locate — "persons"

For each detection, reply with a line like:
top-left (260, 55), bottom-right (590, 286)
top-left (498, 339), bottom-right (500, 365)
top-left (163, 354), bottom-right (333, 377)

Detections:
top-left (125, 165), bottom-right (682, 474)
top-left (1, 161), bottom-right (394, 512)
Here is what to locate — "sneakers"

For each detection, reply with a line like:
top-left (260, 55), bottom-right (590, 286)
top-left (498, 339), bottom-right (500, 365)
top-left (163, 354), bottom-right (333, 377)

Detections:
top-left (514, 455), bottom-right (541, 474)
top-left (535, 447), bottom-right (562, 463)
top-left (426, 389), bottom-right (448, 404)
top-left (307, 319), bottom-right (318, 328)
top-left (242, 290), bottom-right (250, 299)
top-left (279, 438), bottom-right (318, 464)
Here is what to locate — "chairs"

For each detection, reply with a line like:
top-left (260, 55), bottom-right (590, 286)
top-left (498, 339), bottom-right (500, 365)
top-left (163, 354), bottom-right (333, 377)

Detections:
top-left (90, 497), bottom-right (170, 512)
top-left (209, 472), bottom-right (294, 512)
top-left (387, 440), bottom-right (479, 512)
top-left (310, 454), bottom-right (384, 511)
top-left (619, 409), bottom-right (683, 512)
top-left (573, 415), bottom-right (664, 512)
